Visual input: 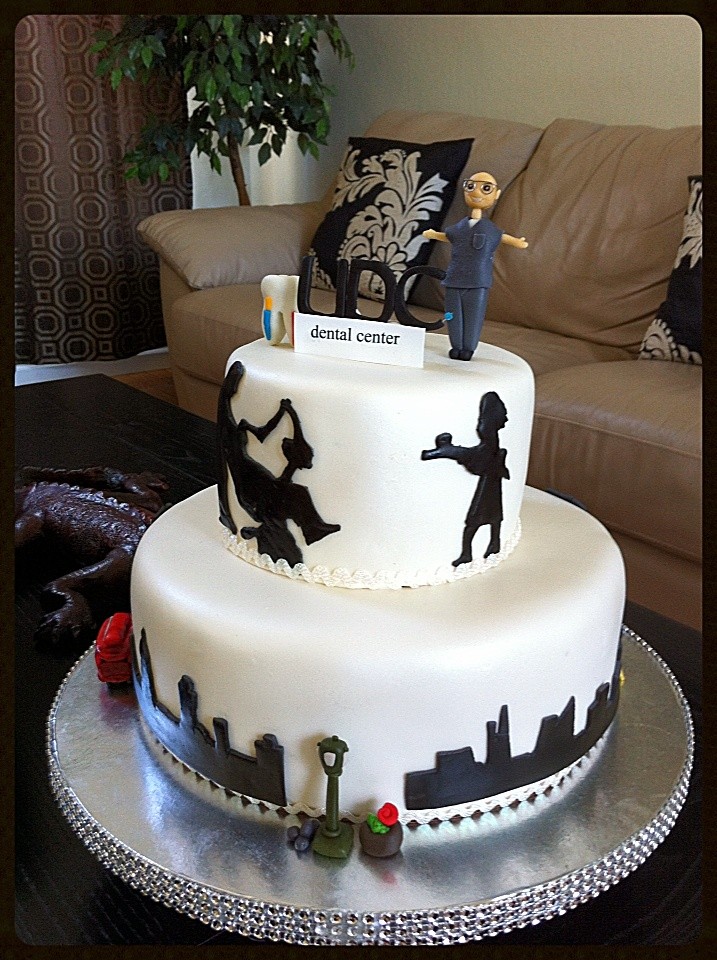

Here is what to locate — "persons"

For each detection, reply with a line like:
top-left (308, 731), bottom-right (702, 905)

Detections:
top-left (423, 172), bottom-right (528, 361)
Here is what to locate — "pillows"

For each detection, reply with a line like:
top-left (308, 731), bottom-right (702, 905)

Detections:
top-left (308, 137), bottom-right (475, 305)
top-left (636, 175), bottom-right (703, 366)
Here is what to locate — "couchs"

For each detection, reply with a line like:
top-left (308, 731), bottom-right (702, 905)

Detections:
top-left (136, 108), bottom-right (703, 634)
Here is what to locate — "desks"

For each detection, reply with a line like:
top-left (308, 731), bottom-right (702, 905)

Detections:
top-left (18, 371), bottom-right (702, 945)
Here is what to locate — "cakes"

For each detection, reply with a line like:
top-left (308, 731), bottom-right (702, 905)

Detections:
top-left (129, 172), bottom-right (624, 826)
